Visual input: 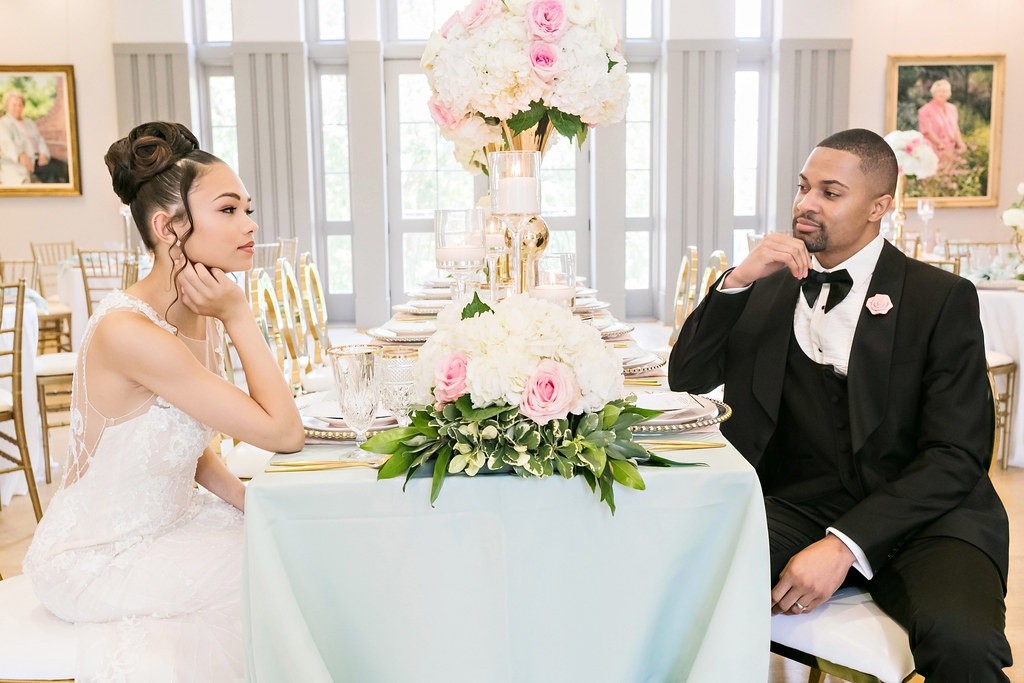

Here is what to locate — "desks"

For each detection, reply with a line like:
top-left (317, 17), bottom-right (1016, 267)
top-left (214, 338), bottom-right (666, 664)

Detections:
top-left (922, 256), bottom-right (1024, 469)
top-left (62, 252), bottom-right (245, 353)
top-left (0, 287), bottom-right (39, 506)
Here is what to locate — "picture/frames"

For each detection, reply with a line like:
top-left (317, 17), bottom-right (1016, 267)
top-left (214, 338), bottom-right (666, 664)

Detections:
top-left (0, 65), bottom-right (83, 196)
top-left (885, 51), bottom-right (1007, 208)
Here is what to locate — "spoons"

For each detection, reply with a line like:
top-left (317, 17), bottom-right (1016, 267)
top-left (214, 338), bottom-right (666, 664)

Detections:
top-left (264, 455), bottom-right (392, 473)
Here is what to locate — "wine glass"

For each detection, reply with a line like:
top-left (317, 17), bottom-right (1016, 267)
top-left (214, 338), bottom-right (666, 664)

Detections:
top-left (917, 199), bottom-right (934, 260)
top-left (488, 150), bottom-right (542, 297)
top-left (327, 343), bottom-right (384, 465)
top-left (383, 346), bottom-right (419, 428)
top-left (434, 208), bottom-right (486, 300)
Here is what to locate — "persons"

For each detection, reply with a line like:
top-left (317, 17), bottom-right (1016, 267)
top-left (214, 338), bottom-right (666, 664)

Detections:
top-left (0, 120), bottom-right (327, 683)
top-left (0, 91), bottom-right (68, 184)
top-left (918, 79), bottom-right (967, 172)
top-left (670, 129), bottom-right (1013, 683)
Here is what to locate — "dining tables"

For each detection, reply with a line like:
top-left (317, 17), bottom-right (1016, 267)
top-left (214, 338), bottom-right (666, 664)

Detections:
top-left (245, 272), bottom-right (756, 683)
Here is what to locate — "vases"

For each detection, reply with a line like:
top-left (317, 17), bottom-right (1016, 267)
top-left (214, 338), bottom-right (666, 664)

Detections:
top-left (480, 149), bottom-right (549, 294)
top-left (891, 174), bottom-right (907, 252)
top-left (1015, 260), bottom-right (1024, 280)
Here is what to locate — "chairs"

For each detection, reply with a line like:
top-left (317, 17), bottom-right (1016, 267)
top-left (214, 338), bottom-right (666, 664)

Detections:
top-left (910, 256), bottom-right (1017, 471)
top-left (771, 355), bottom-right (1000, 683)
top-left (881, 238), bottom-right (924, 260)
top-left (944, 239), bottom-right (1016, 265)
top-left (0, 206), bottom-right (327, 683)
top-left (651, 246), bottom-right (697, 374)
top-left (696, 250), bottom-right (727, 307)
top-left (747, 233), bottom-right (766, 253)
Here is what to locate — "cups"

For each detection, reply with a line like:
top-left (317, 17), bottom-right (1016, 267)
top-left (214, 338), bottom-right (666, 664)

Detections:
top-left (525, 252), bottom-right (576, 314)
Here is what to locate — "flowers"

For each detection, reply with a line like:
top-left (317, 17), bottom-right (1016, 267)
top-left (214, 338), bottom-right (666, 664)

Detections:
top-left (883, 128), bottom-right (940, 180)
top-left (1002, 182), bottom-right (1024, 260)
top-left (977, 266), bottom-right (996, 285)
top-left (419, 0), bottom-right (624, 168)
top-left (361, 293), bottom-right (708, 514)
top-left (866, 294), bottom-right (893, 314)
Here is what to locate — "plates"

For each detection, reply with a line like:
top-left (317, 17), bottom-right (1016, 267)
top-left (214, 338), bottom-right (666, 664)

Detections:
top-left (628, 395), bottom-right (734, 433)
top-left (600, 322), bottom-right (635, 338)
top-left (574, 288), bottom-right (611, 312)
top-left (307, 398), bottom-right (396, 428)
top-left (293, 390), bottom-right (398, 439)
top-left (364, 276), bottom-right (452, 342)
top-left (622, 351), bottom-right (666, 375)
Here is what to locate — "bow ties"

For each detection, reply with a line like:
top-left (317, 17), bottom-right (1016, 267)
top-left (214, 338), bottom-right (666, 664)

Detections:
top-left (801, 269), bottom-right (853, 314)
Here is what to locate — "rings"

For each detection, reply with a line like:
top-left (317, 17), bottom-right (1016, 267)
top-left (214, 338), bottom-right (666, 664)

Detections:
top-left (795, 602), bottom-right (808, 612)
